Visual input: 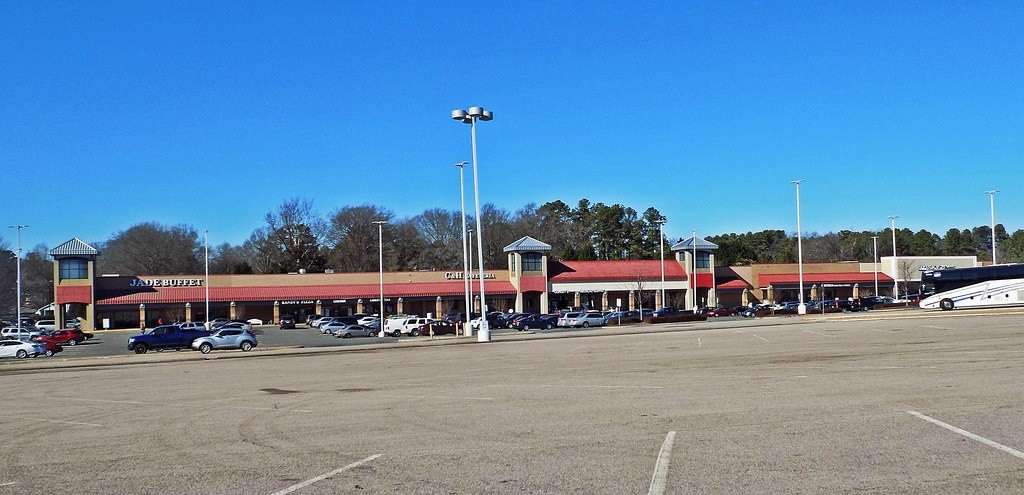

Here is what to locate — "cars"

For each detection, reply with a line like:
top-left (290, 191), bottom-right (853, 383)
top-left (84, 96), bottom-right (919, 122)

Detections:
top-left (279, 315), bottom-right (296, 330)
top-left (306, 296), bottom-right (892, 336)
top-left (0, 340), bottom-right (40, 359)
top-left (172, 317), bottom-right (253, 336)
top-left (333, 326), bottom-right (378, 339)
top-left (192, 328), bottom-right (258, 352)
top-left (0, 317), bottom-right (95, 357)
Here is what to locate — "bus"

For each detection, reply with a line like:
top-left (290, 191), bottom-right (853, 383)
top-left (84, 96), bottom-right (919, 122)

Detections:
top-left (917, 263), bottom-right (1024, 311)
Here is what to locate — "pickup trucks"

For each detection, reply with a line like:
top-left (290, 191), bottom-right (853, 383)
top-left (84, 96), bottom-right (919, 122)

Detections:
top-left (128, 325), bottom-right (210, 355)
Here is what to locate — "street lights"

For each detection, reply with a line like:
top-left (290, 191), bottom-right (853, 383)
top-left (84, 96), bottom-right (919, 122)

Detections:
top-left (984, 189), bottom-right (1000, 265)
top-left (204, 229), bottom-right (211, 331)
top-left (371, 221), bottom-right (389, 338)
top-left (455, 160), bottom-right (471, 322)
top-left (689, 229), bottom-right (698, 314)
top-left (654, 220), bottom-right (665, 308)
top-left (789, 179), bottom-right (807, 314)
top-left (871, 235), bottom-right (880, 297)
top-left (887, 215), bottom-right (902, 304)
top-left (8, 224), bottom-right (30, 341)
top-left (452, 107), bottom-right (493, 343)
